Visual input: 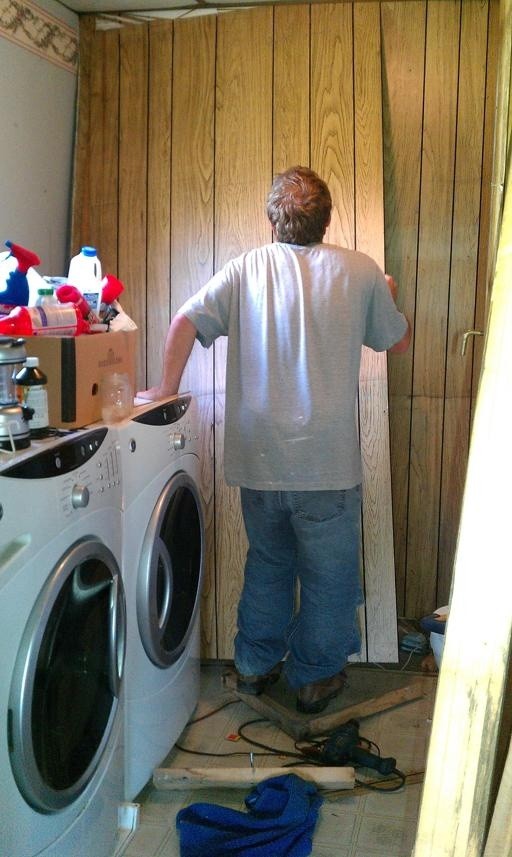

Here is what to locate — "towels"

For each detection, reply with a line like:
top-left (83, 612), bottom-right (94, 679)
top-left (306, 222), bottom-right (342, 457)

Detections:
top-left (175, 774), bottom-right (324, 857)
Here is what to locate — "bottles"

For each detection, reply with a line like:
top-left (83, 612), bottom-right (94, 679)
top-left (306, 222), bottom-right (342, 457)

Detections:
top-left (16, 356), bottom-right (50, 438)
top-left (1, 276), bottom-right (126, 339)
top-left (11, 362), bottom-right (22, 403)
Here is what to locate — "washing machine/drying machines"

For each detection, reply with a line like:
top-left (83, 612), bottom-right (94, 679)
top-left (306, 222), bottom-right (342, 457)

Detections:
top-left (85, 389), bottom-right (206, 804)
top-left (0, 423), bottom-right (127, 857)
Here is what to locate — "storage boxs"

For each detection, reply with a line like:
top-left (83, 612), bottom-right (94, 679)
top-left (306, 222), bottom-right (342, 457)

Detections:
top-left (8, 330), bottom-right (137, 431)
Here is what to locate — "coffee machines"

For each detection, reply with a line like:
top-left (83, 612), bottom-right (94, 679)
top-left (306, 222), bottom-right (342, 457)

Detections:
top-left (1, 337), bottom-right (35, 452)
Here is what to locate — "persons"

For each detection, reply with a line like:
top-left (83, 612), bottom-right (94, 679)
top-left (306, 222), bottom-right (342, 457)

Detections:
top-left (136, 165), bottom-right (411, 715)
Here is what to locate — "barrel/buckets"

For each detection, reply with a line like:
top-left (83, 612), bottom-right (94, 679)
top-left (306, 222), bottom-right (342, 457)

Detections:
top-left (66, 245), bottom-right (103, 318)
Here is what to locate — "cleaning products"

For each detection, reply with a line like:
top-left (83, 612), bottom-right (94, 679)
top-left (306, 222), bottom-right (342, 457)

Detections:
top-left (0, 238), bottom-right (41, 320)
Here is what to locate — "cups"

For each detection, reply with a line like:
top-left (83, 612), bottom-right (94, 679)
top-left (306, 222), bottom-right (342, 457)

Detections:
top-left (102, 371), bottom-right (133, 426)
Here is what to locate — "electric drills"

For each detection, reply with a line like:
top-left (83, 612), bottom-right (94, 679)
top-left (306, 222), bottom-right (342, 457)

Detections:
top-left (323, 712), bottom-right (396, 776)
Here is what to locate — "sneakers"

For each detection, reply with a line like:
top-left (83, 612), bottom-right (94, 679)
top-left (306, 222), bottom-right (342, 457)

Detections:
top-left (296, 672), bottom-right (344, 715)
top-left (236, 669), bottom-right (280, 695)
